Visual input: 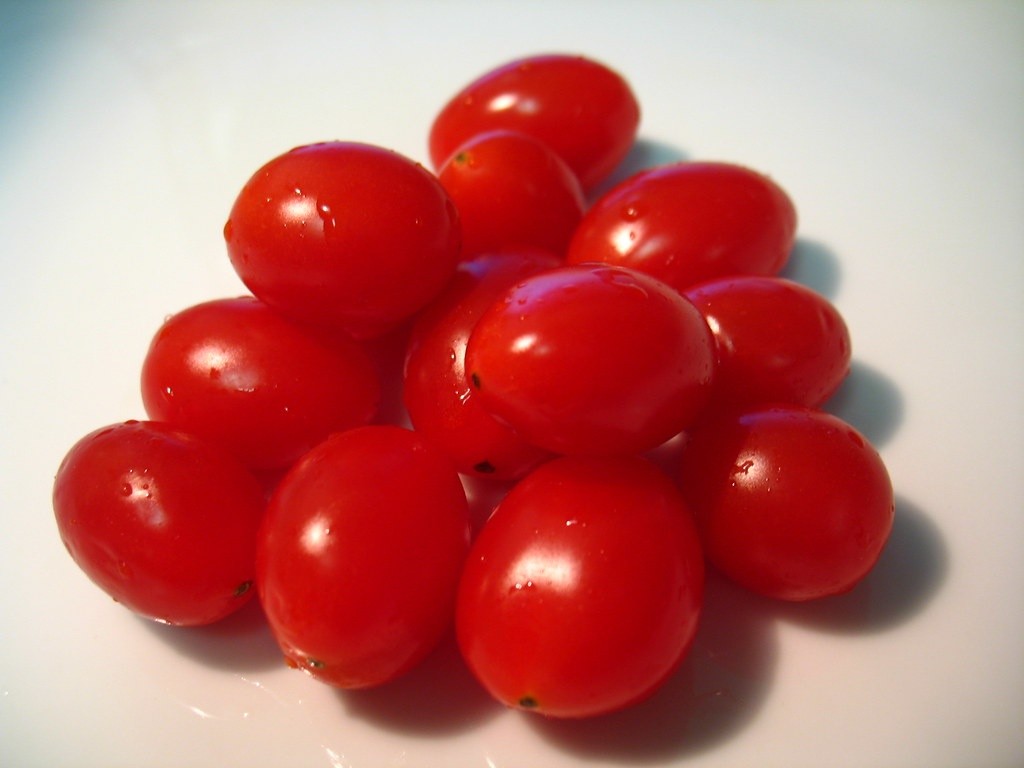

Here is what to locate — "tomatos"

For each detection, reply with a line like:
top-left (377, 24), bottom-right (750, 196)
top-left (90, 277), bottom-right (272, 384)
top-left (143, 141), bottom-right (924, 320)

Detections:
top-left (53, 51), bottom-right (895, 719)
